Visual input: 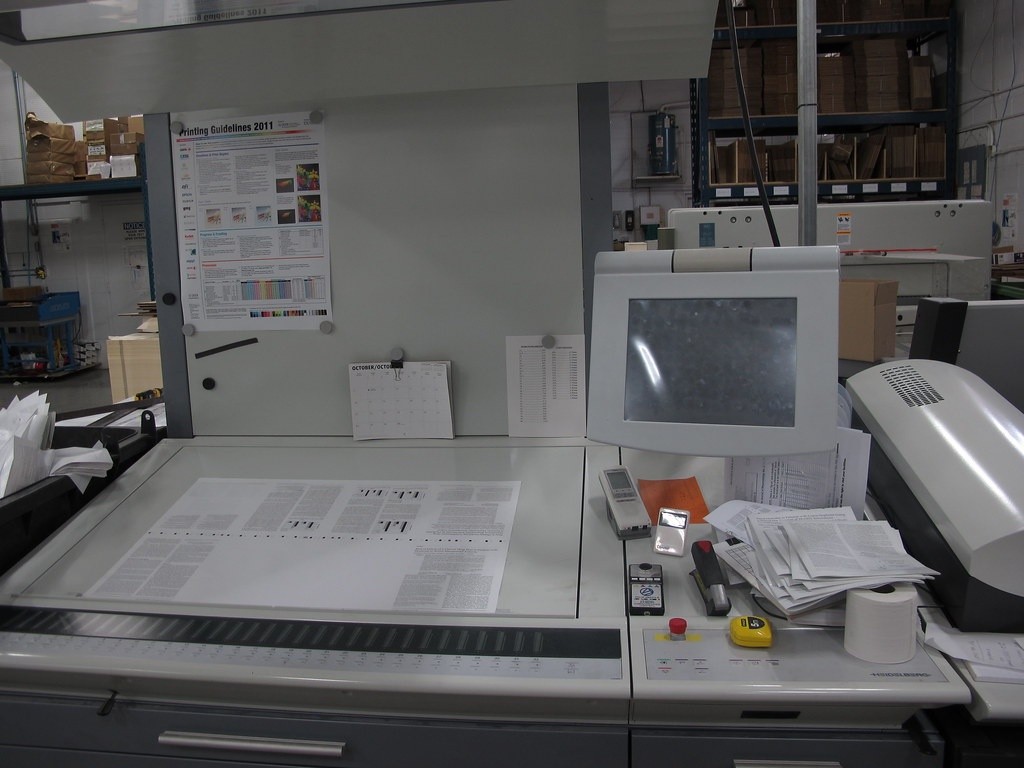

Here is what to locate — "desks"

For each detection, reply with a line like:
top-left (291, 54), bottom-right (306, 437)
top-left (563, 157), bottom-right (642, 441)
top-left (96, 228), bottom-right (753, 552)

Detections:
top-left (859, 476), bottom-right (1024, 728)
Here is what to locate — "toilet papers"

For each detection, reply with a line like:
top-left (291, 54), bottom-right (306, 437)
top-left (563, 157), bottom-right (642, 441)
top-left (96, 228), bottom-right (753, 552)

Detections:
top-left (842, 580), bottom-right (921, 667)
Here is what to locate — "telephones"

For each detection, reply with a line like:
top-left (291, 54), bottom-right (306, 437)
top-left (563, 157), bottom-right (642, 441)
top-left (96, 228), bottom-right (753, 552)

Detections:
top-left (597, 464), bottom-right (651, 540)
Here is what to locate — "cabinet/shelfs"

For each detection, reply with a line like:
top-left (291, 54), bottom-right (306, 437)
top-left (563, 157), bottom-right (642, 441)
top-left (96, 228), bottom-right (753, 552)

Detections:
top-left (1, 678), bottom-right (947, 768)
top-left (688, 0), bottom-right (961, 209)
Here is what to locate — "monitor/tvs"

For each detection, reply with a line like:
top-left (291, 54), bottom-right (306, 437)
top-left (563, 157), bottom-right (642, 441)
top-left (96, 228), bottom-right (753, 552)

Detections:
top-left (587, 248), bottom-right (838, 459)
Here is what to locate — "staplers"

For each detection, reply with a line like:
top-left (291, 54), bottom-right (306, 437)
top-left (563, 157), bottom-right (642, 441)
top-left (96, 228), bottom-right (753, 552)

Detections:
top-left (689, 540), bottom-right (732, 617)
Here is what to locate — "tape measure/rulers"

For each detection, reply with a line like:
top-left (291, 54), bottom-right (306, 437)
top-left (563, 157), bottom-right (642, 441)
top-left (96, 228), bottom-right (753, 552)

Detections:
top-left (730, 615), bottom-right (772, 648)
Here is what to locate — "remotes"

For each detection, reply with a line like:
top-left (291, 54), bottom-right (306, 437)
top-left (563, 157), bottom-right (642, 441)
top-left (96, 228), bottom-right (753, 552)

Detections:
top-left (599, 464), bottom-right (651, 539)
